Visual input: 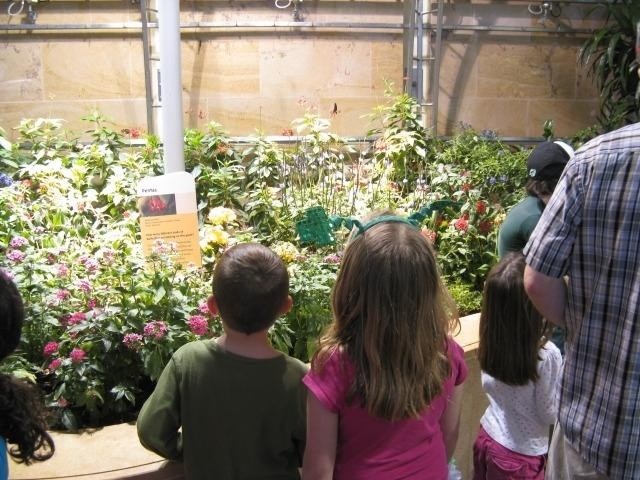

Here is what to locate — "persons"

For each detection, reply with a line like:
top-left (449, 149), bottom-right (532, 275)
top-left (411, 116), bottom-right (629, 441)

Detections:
top-left (497, 141), bottom-right (575, 359)
top-left (302, 221), bottom-right (467, 480)
top-left (474, 253), bottom-right (564, 480)
top-left (0, 268), bottom-right (54, 480)
top-left (522, 122), bottom-right (640, 480)
top-left (137, 243), bottom-right (308, 480)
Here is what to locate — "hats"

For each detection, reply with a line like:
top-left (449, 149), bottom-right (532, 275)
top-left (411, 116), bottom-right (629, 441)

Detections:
top-left (527, 141), bottom-right (574, 181)
